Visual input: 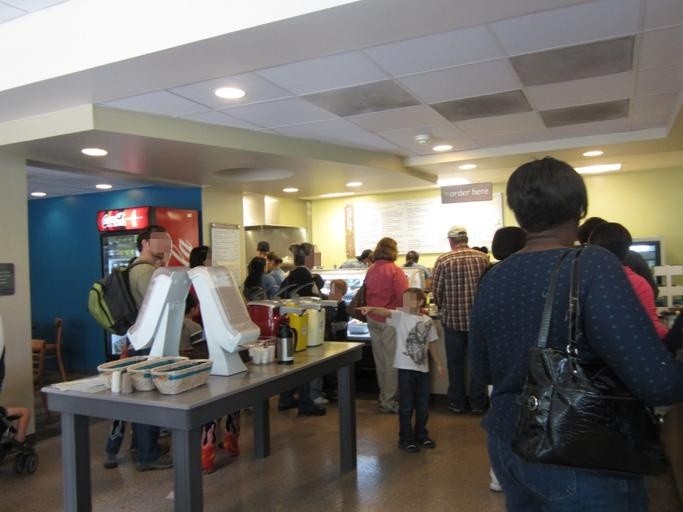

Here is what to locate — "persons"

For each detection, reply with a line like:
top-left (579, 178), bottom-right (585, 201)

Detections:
top-left (0, 407), bottom-right (32, 451)
top-left (469, 156), bottom-right (683, 511)
top-left (157, 217), bottom-right (680, 476)
top-left (101, 224), bottom-right (175, 471)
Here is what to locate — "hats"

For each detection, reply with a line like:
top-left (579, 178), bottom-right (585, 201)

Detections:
top-left (445, 226), bottom-right (467, 237)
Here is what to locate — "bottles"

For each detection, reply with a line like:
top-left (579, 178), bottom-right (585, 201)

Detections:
top-left (106, 234), bottom-right (137, 267)
top-left (428, 298), bottom-right (438, 316)
top-left (275, 322), bottom-right (297, 365)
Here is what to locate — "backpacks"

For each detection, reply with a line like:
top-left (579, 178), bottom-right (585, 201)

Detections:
top-left (88, 256), bottom-right (153, 336)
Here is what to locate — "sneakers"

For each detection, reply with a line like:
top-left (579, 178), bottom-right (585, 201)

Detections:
top-left (490, 467), bottom-right (502, 490)
top-left (12, 438), bottom-right (36, 452)
top-left (278, 395), bottom-right (490, 453)
top-left (104, 428), bottom-right (173, 471)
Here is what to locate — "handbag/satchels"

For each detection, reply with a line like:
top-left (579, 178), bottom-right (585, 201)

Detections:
top-left (344, 284), bottom-right (368, 323)
top-left (512, 345), bottom-right (672, 475)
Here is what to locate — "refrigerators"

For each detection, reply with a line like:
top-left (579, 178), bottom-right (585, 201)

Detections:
top-left (96, 205), bottom-right (202, 361)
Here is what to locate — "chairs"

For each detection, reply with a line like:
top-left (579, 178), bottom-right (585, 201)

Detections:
top-left (31, 340), bottom-right (52, 420)
top-left (40, 318), bottom-right (68, 383)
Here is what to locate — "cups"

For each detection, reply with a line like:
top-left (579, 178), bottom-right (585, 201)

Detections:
top-left (121, 372), bottom-right (132, 394)
top-left (250, 346), bottom-right (275, 365)
top-left (109, 371), bottom-right (120, 393)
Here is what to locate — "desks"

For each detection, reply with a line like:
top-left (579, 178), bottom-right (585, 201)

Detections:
top-left (43, 343), bottom-right (363, 512)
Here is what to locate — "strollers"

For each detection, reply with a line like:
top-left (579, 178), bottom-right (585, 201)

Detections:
top-left (0, 412), bottom-right (41, 477)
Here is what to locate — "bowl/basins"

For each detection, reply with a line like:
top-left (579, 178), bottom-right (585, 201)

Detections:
top-left (345, 322), bottom-right (369, 333)
